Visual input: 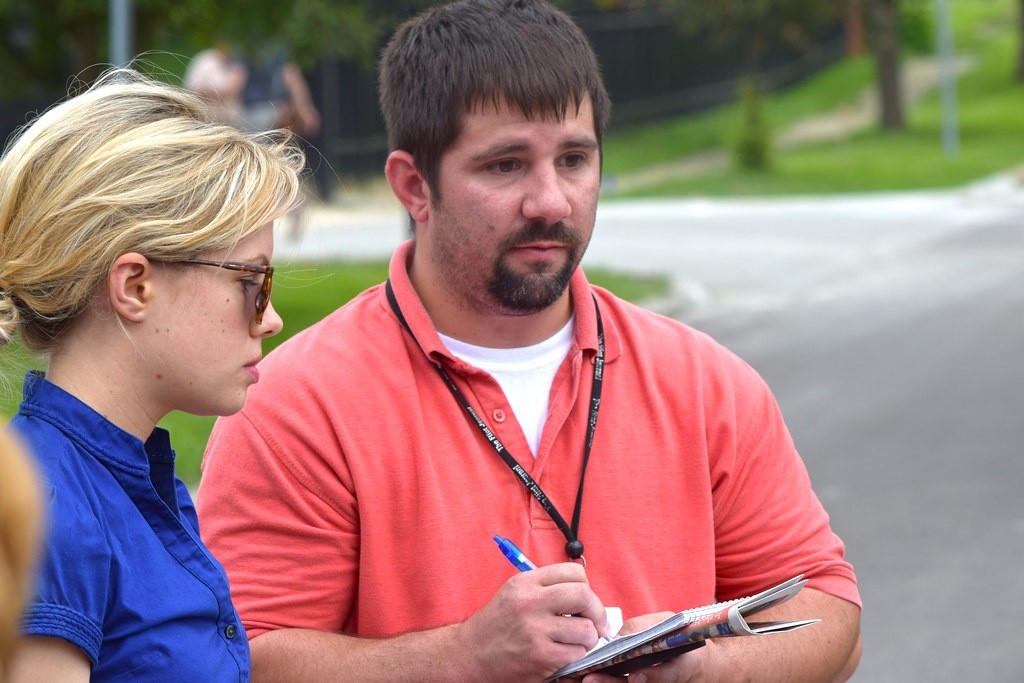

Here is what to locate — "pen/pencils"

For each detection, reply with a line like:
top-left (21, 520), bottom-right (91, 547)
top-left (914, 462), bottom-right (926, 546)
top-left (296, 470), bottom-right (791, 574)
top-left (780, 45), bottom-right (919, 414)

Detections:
top-left (493, 534), bottom-right (615, 644)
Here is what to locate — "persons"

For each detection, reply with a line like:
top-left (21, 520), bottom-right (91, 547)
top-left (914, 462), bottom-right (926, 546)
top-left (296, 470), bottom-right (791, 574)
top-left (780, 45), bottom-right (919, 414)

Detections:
top-left (195, 0), bottom-right (863, 683)
top-left (0, 66), bottom-right (305, 683)
top-left (184, 46), bottom-right (320, 145)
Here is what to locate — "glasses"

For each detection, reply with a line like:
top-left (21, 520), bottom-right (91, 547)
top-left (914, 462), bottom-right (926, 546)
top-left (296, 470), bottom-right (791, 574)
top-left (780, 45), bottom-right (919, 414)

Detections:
top-left (148, 255), bottom-right (277, 324)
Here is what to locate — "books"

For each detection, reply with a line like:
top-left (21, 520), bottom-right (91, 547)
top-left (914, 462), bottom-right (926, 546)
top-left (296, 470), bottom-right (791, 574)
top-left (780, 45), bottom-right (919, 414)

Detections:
top-left (547, 596), bottom-right (749, 671)
